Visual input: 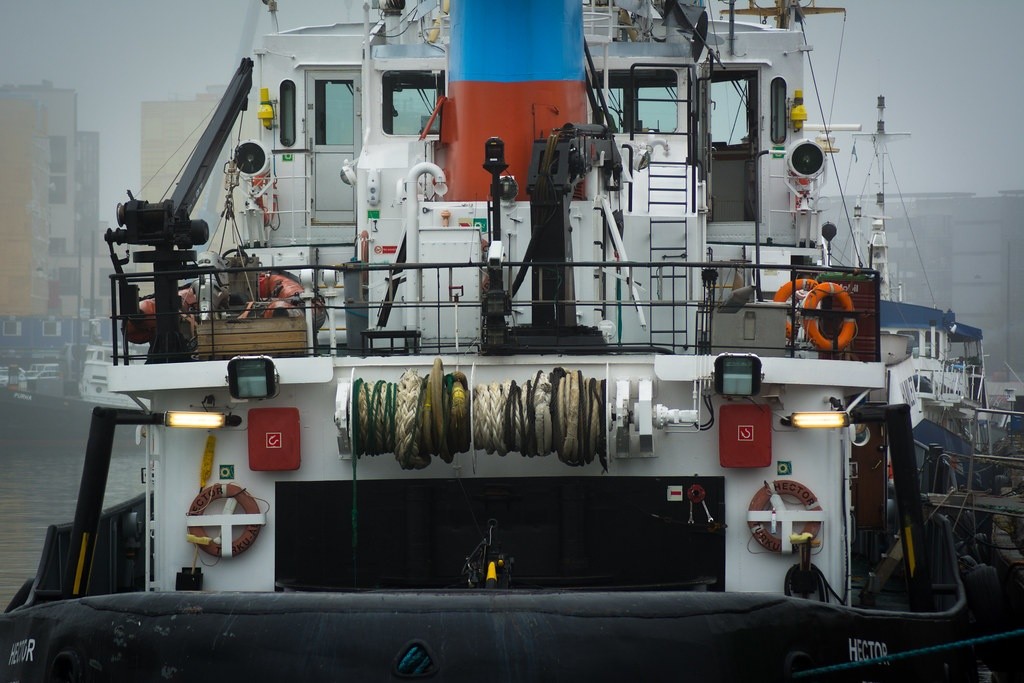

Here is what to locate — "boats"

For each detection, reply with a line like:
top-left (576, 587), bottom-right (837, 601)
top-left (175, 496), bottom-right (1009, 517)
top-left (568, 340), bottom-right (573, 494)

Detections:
top-left (0, 0), bottom-right (967, 683)
top-left (845, 94), bottom-right (1014, 494)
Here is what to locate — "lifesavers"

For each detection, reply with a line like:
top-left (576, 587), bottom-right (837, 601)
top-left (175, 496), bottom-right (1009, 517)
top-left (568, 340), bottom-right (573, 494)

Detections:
top-left (187, 483), bottom-right (261, 559)
top-left (773, 278), bottom-right (818, 342)
top-left (748, 479), bottom-right (821, 553)
top-left (802, 282), bottom-right (855, 350)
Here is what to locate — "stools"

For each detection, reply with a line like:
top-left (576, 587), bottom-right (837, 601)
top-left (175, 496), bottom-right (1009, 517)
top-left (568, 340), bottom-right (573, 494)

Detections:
top-left (362, 331), bottom-right (422, 355)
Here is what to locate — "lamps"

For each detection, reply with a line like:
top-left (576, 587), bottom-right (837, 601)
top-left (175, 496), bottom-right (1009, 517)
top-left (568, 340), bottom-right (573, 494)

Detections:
top-left (711, 353), bottom-right (765, 397)
top-left (340, 165), bottom-right (356, 187)
top-left (227, 355), bottom-right (280, 401)
top-left (780, 411), bottom-right (850, 430)
top-left (500, 176), bottom-right (520, 207)
top-left (634, 148), bottom-right (652, 172)
top-left (163, 410), bottom-right (242, 428)
